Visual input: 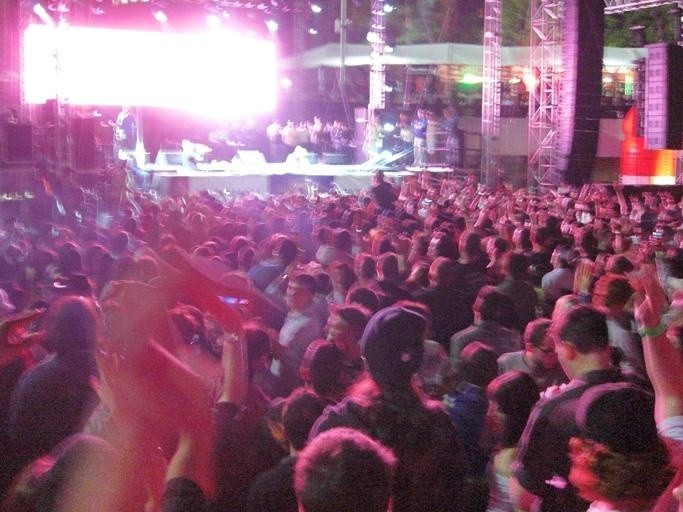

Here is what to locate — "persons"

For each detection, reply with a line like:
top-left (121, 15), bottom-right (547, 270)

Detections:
top-left (0, 106), bottom-right (682, 511)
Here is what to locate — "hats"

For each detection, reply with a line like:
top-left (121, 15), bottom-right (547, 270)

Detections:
top-left (576, 382), bottom-right (659, 453)
top-left (31, 272), bottom-right (93, 300)
top-left (301, 340), bottom-right (341, 382)
top-left (360, 307), bottom-right (427, 378)
